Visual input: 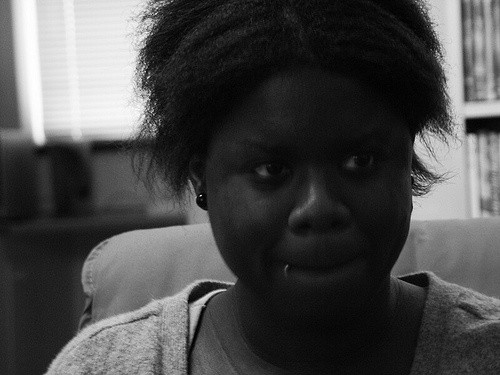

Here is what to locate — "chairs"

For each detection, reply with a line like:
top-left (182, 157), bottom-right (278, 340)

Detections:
top-left (78, 218), bottom-right (500, 336)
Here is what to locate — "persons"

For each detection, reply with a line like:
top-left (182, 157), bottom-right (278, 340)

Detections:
top-left (46, 1), bottom-right (500, 375)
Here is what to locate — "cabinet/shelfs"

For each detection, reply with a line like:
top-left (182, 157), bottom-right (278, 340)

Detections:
top-left (458, 0), bottom-right (500, 216)
